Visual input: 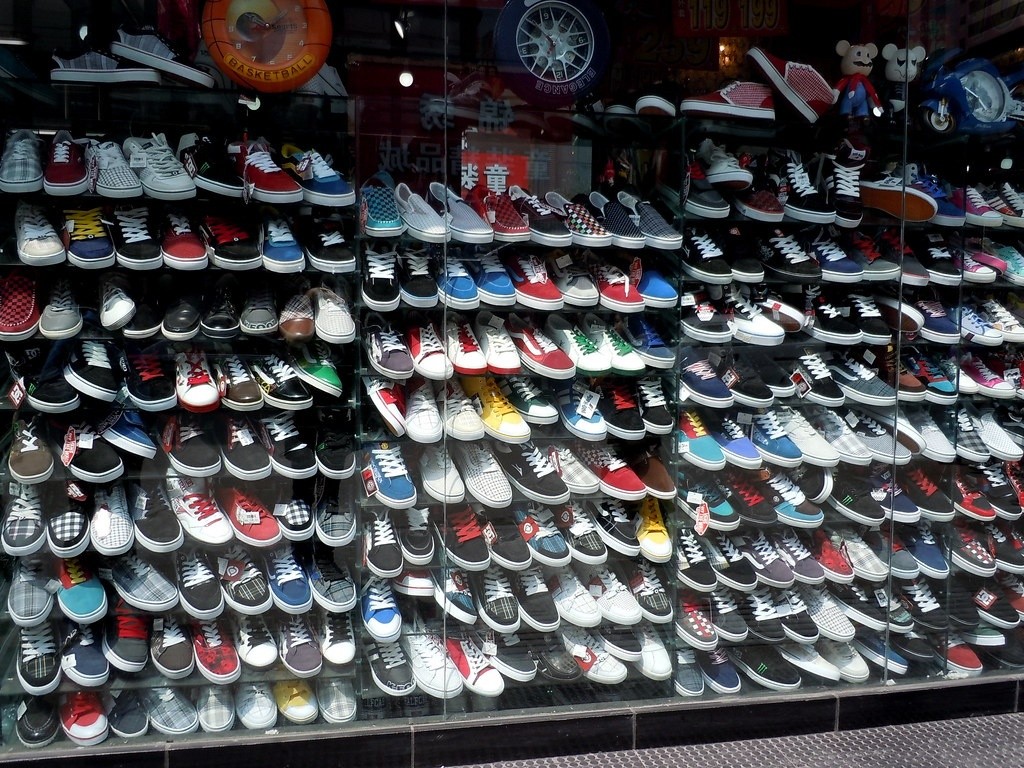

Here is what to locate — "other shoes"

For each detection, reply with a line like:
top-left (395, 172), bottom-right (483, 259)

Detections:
top-left (696, 117), bottom-right (777, 138)
top-left (503, 95), bottom-right (680, 131)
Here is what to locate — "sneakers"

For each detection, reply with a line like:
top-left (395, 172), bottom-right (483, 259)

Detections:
top-left (2, 30), bottom-right (360, 125)
top-left (680, 80), bottom-right (775, 123)
top-left (0, 123), bottom-right (1021, 751)
top-left (429, 69), bottom-right (512, 122)
top-left (746, 46), bottom-right (835, 124)
